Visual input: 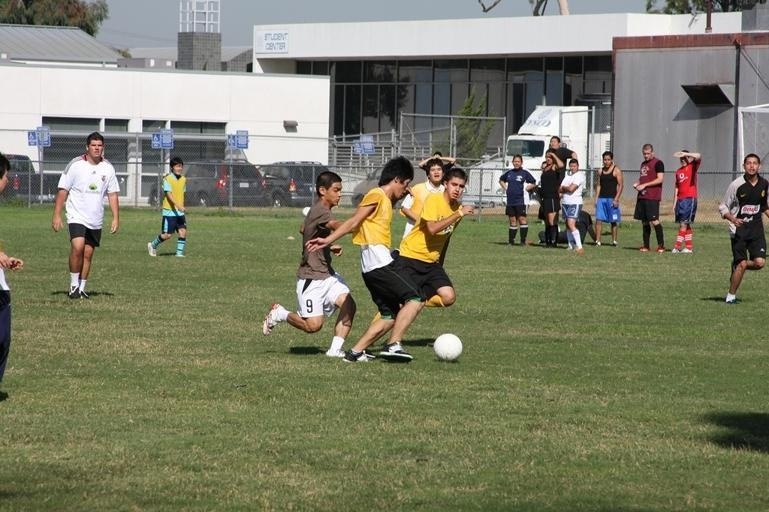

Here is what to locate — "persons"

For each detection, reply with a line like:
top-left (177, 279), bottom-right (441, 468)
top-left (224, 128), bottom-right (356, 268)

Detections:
top-left (51, 131), bottom-right (121, 300)
top-left (499, 154), bottom-right (536, 250)
top-left (0, 150), bottom-right (23, 403)
top-left (306, 152), bottom-right (473, 362)
top-left (594, 150), bottom-right (623, 249)
top-left (148, 157), bottom-right (188, 258)
top-left (633, 143), bottom-right (665, 252)
top-left (538, 135), bottom-right (596, 257)
top-left (672, 149), bottom-right (701, 253)
top-left (261, 171), bottom-right (356, 357)
top-left (718, 153), bottom-right (769, 303)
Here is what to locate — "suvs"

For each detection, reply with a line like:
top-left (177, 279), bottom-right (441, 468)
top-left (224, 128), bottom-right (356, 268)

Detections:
top-left (258, 161), bottom-right (330, 205)
top-left (148, 161), bottom-right (270, 208)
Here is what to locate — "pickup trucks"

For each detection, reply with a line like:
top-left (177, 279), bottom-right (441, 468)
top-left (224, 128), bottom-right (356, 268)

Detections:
top-left (1, 153), bottom-right (70, 204)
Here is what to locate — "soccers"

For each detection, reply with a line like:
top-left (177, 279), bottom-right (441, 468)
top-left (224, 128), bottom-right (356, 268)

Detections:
top-left (434, 333), bottom-right (462, 359)
top-left (303, 207), bottom-right (311, 216)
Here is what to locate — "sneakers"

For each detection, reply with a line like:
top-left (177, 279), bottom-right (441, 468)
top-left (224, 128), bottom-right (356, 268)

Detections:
top-left (148, 242), bottom-right (157, 257)
top-left (380, 341), bottom-right (414, 362)
top-left (175, 254), bottom-right (186, 257)
top-left (68, 288), bottom-right (89, 300)
top-left (343, 349), bottom-right (378, 363)
top-left (325, 348), bottom-right (345, 358)
top-left (725, 295), bottom-right (740, 305)
top-left (730, 260), bottom-right (737, 282)
top-left (261, 301), bottom-right (284, 336)
top-left (505, 240), bottom-right (695, 255)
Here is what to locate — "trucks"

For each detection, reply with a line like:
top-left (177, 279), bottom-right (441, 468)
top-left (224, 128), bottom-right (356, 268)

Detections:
top-left (503, 105), bottom-right (609, 195)
